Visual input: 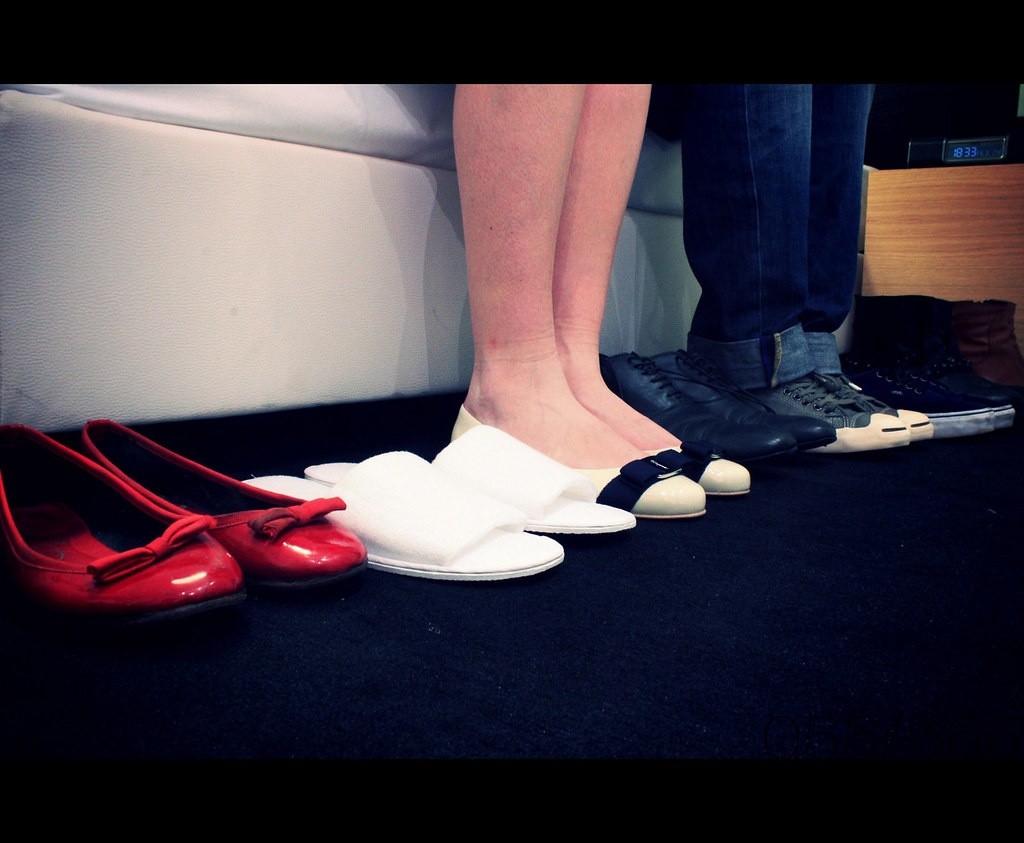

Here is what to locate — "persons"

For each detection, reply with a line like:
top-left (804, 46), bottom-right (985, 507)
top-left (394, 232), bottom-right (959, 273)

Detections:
top-left (453, 85), bottom-right (753, 519)
top-left (680, 85), bottom-right (935, 453)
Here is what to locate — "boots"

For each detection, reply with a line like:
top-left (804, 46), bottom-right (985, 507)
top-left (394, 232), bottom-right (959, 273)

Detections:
top-left (854, 294), bottom-right (1024, 415)
top-left (952, 300), bottom-right (1024, 388)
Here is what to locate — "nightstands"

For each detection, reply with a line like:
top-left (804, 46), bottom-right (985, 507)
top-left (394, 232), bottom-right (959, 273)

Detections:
top-left (861, 164), bottom-right (1024, 356)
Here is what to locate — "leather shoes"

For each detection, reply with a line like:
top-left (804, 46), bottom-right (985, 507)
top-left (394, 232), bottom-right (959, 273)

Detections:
top-left (643, 439), bottom-right (751, 495)
top-left (599, 352), bottom-right (798, 466)
top-left (650, 348), bottom-right (837, 463)
top-left (82, 418), bottom-right (369, 588)
top-left (451, 404), bottom-right (706, 519)
top-left (0, 421), bottom-right (248, 624)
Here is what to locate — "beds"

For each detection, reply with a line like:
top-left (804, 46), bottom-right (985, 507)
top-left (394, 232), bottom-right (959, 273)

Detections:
top-left (0, 85), bottom-right (872, 431)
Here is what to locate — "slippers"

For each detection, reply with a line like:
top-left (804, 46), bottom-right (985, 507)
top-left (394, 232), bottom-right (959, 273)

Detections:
top-left (239, 451), bottom-right (564, 581)
top-left (303, 424), bottom-right (636, 535)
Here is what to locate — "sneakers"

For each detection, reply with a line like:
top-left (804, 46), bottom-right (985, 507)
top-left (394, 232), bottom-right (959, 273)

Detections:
top-left (813, 369), bottom-right (935, 443)
top-left (890, 366), bottom-right (1016, 429)
top-left (750, 376), bottom-right (910, 452)
top-left (844, 364), bottom-right (996, 439)
